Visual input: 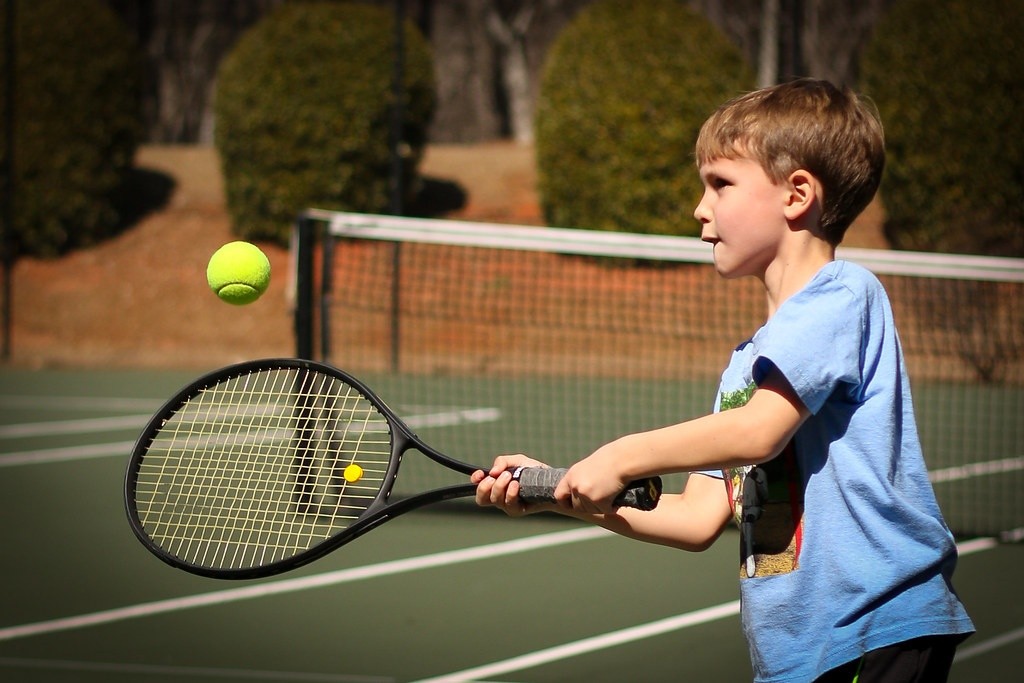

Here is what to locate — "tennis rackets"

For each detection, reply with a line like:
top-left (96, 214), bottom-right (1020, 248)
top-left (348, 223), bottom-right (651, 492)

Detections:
top-left (120, 354), bottom-right (664, 583)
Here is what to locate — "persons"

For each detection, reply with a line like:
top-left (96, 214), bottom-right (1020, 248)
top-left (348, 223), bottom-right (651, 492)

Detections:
top-left (470, 79), bottom-right (977, 683)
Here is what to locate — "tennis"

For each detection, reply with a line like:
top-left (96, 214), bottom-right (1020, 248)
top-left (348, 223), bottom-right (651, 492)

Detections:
top-left (205, 239), bottom-right (273, 306)
